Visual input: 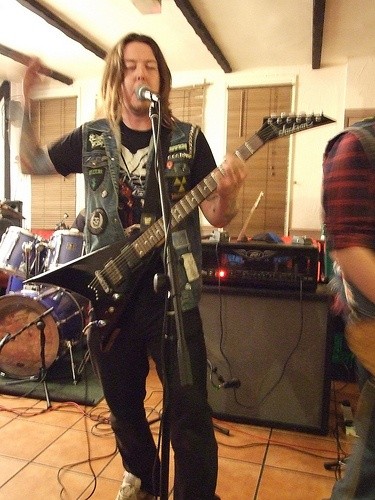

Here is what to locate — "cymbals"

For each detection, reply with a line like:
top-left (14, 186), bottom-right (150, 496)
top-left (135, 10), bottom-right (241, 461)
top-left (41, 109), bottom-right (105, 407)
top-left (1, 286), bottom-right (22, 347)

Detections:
top-left (0, 203), bottom-right (25, 220)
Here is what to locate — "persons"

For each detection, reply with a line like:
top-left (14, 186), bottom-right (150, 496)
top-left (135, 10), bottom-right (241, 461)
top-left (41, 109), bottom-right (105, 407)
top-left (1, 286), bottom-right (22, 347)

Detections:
top-left (321, 114), bottom-right (375, 500)
top-left (19, 35), bottom-right (251, 500)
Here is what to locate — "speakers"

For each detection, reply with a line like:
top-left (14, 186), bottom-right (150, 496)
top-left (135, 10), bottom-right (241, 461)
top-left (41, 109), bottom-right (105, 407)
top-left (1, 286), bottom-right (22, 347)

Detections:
top-left (197, 285), bottom-right (334, 436)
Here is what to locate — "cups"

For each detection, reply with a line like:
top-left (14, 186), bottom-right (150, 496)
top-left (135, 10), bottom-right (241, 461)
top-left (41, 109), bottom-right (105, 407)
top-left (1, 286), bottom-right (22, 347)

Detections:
top-left (220, 231), bottom-right (228, 242)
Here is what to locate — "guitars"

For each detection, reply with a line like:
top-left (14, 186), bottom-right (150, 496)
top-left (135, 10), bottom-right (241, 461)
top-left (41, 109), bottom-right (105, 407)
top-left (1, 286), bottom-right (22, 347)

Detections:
top-left (19, 112), bottom-right (337, 351)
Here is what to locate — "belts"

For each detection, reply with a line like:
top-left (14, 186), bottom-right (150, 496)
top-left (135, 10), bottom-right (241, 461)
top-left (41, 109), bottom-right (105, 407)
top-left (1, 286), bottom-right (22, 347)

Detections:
top-left (136, 274), bottom-right (170, 294)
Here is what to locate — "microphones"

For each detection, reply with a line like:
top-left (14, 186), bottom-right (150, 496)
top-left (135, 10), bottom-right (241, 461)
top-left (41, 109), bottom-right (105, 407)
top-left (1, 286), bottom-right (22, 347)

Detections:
top-left (135, 84), bottom-right (161, 101)
top-left (0, 332), bottom-right (11, 352)
top-left (219, 378), bottom-right (242, 389)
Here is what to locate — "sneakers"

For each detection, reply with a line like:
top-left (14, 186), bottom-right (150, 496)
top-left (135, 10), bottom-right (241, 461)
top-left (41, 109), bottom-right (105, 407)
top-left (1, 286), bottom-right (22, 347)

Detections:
top-left (117, 471), bottom-right (155, 500)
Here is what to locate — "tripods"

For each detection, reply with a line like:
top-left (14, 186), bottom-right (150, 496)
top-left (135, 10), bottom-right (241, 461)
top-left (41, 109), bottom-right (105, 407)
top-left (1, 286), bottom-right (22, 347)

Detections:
top-left (0, 306), bottom-right (71, 408)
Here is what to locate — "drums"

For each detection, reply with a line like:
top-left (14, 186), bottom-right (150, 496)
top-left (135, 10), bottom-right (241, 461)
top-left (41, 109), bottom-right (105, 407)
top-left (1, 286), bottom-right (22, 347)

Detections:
top-left (46, 229), bottom-right (85, 272)
top-left (0, 284), bottom-right (84, 380)
top-left (0, 225), bottom-right (49, 278)
top-left (5, 274), bottom-right (24, 296)
